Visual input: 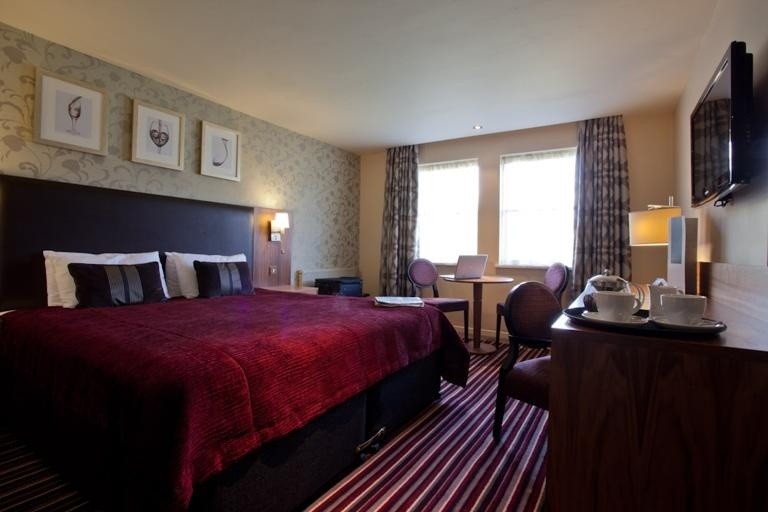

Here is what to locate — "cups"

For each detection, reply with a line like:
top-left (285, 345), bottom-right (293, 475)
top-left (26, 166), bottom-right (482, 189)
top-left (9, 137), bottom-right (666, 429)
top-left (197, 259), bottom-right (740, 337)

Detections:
top-left (659, 293), bottom-right (706, 324)
top-left (647, 285), bottom-right (686, 318)
top-left (294, 265), bottom-right (303, 289)
top-left (593, 291), bottom-right (642, 319)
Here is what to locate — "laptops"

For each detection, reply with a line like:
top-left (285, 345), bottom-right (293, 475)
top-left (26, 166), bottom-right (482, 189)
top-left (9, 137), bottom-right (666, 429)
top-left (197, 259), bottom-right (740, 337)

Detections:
top-left (439, 255), bottom-right (488, 279)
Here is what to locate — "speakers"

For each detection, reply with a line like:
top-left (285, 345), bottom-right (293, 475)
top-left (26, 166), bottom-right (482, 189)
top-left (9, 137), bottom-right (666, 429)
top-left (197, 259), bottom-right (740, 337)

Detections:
top-left (668, 217), bottom-right (697, 295)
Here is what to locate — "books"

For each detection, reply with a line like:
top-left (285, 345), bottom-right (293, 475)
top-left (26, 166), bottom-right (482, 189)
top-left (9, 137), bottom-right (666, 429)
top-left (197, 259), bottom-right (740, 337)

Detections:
top-left (374, 296), bottom-right (424, 308)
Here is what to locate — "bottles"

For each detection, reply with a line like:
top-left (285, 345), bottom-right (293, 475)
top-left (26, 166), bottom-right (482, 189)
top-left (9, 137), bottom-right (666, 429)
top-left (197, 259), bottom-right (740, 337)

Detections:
top-left (669, 195), bottom-right (673, 207)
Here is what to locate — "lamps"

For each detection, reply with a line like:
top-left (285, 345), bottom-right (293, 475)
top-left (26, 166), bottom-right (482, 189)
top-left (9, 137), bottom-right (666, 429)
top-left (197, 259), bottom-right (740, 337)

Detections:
top-left (269, 211), bottom-right (288, 236)
top-left (626, 195), bottom-right (682, 247)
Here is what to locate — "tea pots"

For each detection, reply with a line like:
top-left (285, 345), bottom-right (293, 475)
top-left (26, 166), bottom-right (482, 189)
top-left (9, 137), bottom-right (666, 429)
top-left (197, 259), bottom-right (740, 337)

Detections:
top-left (580, 269), bottom-right (645, 310)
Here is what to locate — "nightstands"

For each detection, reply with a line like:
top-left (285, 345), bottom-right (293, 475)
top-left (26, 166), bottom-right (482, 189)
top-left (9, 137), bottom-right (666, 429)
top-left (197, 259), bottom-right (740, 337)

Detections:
top-left (257, 284), bottom-right (317, 295)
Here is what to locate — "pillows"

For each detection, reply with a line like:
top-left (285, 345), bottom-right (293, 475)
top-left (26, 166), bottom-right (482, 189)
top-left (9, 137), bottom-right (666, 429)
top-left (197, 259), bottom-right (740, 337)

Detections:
top-left (40, 249), bottom-right (63, 307)
top-left (193, 260), bottom-right (255, 298)
top-left (67, 261), bottom-right (166, 308)
top-left (50, 251), bottom-right (169, 307)
top-left (173, 252), bottom-right (246, 301)
top-left (163, 252), bottom-right (182, 295)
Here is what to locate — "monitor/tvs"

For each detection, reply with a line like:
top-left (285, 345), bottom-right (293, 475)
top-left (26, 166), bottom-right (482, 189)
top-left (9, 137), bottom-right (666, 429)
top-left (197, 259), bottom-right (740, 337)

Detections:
top-left (690, 41), bottom-right (753, 207)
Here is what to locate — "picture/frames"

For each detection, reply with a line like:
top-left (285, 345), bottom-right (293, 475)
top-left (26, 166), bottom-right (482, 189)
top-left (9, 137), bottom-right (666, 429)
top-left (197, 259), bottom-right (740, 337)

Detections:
top-left (196, 118), bottom-right (242, 183)
top-left (32, 66), bottom-right (109, 158)
top-left (129, 99), bottom-right (185, 173)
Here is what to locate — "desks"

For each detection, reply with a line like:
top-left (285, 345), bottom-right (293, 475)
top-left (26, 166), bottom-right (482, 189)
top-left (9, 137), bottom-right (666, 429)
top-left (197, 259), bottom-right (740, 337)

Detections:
top-left (542, 280), bottom-right (767, 512)
top-left (443, 275), bottom-right (514, 354)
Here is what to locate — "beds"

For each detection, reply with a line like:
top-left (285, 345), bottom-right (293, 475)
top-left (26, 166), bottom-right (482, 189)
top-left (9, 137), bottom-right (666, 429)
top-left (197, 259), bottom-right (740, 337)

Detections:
top-left (0, 173), bottom-right (472, 512)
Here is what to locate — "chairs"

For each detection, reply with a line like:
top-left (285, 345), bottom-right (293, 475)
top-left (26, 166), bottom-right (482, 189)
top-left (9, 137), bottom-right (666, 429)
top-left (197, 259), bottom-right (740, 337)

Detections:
top-left (491, 279), bottom-right (561, 441)
top-left (494, 262), bottom-right (570, 348)
top-left (406, 257), bottom-right (470, 342)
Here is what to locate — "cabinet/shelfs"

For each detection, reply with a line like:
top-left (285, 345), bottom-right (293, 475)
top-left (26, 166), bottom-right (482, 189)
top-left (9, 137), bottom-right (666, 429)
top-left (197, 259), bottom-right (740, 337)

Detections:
top-left (314, 275), bottom-right (362, 296)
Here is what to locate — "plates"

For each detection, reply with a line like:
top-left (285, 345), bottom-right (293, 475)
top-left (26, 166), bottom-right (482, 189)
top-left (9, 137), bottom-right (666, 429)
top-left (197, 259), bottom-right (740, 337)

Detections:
top-left (581, 311), bottom-right (650, 325)
top-left (648, 318), bottom-right (716, 329)
